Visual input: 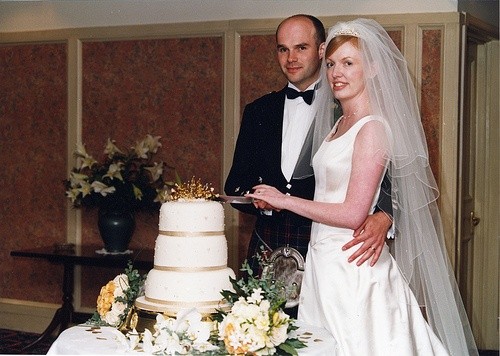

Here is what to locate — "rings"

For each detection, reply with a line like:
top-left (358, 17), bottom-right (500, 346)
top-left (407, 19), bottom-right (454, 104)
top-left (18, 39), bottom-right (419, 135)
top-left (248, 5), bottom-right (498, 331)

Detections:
top-left (370, 246), bottom-right (376, 251)
top-left (257, 191), bottom-right (261, 194)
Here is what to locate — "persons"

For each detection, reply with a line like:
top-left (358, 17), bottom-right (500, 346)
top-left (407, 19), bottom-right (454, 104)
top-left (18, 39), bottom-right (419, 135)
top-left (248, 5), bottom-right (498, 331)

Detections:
top-left (243, 18), bottom-right (479, 356)
top-left (223, 15), bottom-right (401, 319)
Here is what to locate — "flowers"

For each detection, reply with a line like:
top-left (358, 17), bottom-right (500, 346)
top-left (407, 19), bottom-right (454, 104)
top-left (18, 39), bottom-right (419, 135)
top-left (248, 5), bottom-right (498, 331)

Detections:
top-left (62, 137), bottom-right (177, 218)
top-left (88, 251), bottom-right (309, 356)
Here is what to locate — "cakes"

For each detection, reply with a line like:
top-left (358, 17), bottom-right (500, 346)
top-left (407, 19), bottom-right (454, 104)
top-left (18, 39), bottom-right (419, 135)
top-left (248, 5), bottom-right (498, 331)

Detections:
top-left (144, 174), bottom-right (237, 306)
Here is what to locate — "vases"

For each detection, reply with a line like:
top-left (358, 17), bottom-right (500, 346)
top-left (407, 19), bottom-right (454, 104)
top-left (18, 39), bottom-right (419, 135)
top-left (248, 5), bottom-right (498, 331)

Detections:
top-left (97, 206), bottom-right (136, 253)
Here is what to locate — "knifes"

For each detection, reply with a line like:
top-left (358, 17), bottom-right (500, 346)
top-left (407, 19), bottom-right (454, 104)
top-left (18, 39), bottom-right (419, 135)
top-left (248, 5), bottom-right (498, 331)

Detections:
top-left (219, 196), bottom-right (259, 204)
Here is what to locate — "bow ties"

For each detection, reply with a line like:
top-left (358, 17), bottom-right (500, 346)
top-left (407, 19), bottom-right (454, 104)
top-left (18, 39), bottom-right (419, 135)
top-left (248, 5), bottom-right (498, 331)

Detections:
top-left (286, 81), bottom-right (322, 105)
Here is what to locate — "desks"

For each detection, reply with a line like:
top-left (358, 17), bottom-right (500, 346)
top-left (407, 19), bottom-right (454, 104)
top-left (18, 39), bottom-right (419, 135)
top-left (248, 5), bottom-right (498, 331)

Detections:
top-left (46, 319), bottom-right (339, 356)
top-left (10, 244), bottom-right (154, 352)
